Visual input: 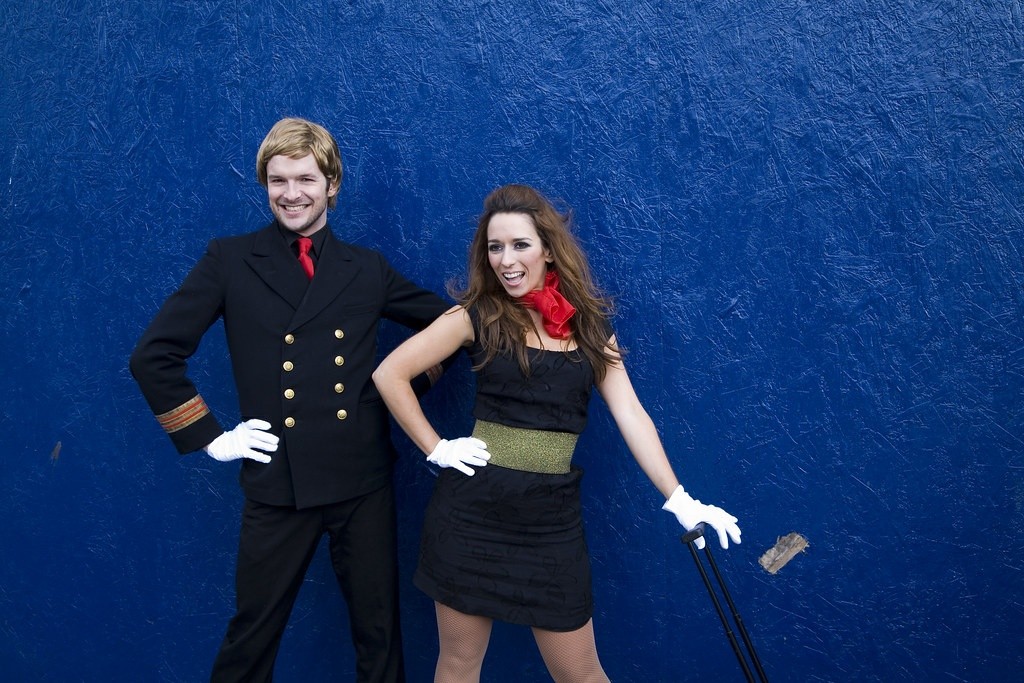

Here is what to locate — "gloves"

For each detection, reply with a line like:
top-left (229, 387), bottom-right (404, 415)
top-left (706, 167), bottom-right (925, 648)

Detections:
top-left (662, 484), bottom-right (741, 549)
top-left (202, 419), bottom-right (279, 463)
top-left (426, 436), bottom-right (491, 476)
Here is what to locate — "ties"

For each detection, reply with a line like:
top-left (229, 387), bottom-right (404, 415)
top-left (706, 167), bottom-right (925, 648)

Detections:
top-left (297, 237), bottom-right (314, 281)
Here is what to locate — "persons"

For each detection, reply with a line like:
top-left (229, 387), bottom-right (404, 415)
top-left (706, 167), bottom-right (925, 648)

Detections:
top-left (371, 182), bottom-right (742, 683)
top-left (129, 118), bottom-right (467, 683)
